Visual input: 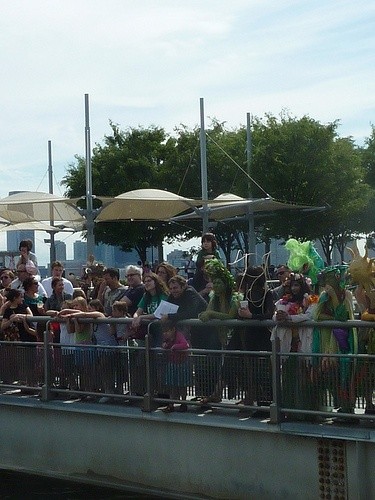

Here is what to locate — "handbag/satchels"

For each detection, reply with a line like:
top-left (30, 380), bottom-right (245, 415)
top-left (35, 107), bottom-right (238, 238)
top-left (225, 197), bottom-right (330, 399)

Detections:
top-left (3, 309), bottom-right (20, 341)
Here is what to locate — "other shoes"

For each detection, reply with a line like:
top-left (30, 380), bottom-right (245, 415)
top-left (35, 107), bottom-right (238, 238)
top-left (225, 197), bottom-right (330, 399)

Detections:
top-left (365, 404), bottom-right (375, 415)
top-left (337, 408), bottom-right (354, 414)
top-left (21, 382), bottom-right (269, 414)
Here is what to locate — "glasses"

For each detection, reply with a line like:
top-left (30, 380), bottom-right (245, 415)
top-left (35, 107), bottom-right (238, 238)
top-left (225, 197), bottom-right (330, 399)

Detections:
top-left (16, 270), bottom-right (26, 273)
top-left (1, 276), bottom-right (8, 280)
top-left (125, 274), bottom-right (137, 277)
top-left (33, 282), bottom-right (38, 285)
top-left (144, 279), bottom-right (153, 284)
top-left (277, 271), bottom-right (288, 276)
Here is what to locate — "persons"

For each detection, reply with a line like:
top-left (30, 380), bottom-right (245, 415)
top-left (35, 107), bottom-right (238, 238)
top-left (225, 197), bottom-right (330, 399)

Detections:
top-left (16, 239), bottom-right (39, 275)
top-left (0, 255), bottom-right (375, 425)
top-left (193, 233), bottom-right (221, 291)
